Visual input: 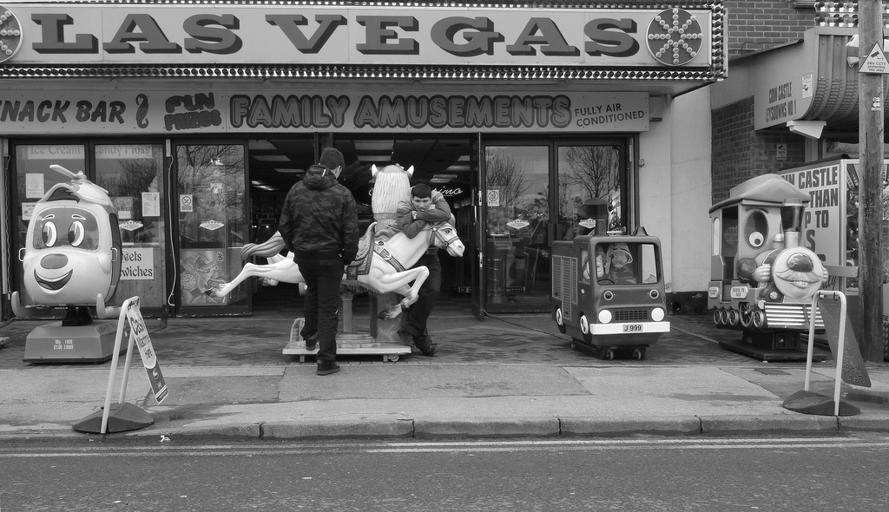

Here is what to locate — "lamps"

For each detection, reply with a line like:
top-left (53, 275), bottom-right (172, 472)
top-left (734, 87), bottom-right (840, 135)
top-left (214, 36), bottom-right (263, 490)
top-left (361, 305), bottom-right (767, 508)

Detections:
top-left (812, 0), bottom-right (857, 25)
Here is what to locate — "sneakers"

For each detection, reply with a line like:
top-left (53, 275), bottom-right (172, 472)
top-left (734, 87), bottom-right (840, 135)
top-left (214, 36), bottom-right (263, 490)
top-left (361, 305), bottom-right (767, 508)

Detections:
top-left (415, 336), bottom-right (435, 355)
top-left (398, 329), bottom-right (414, 345)
top-left (306, 340), bottom-right (315, 350)
top-left (317, 360), bottom-right (339, 375)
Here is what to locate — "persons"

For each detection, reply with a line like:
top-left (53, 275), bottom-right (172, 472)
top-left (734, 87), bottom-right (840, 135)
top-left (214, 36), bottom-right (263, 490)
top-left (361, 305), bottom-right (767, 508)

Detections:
top-left (278, 147), bottom-right (360, 376)
top-left (395, 184), bottom-right (451, 355)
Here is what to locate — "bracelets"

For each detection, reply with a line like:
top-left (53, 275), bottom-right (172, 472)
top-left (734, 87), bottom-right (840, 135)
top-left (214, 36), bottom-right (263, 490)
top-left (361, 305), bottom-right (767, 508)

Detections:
top-left (411, 210), bottom-right (417, 219)
